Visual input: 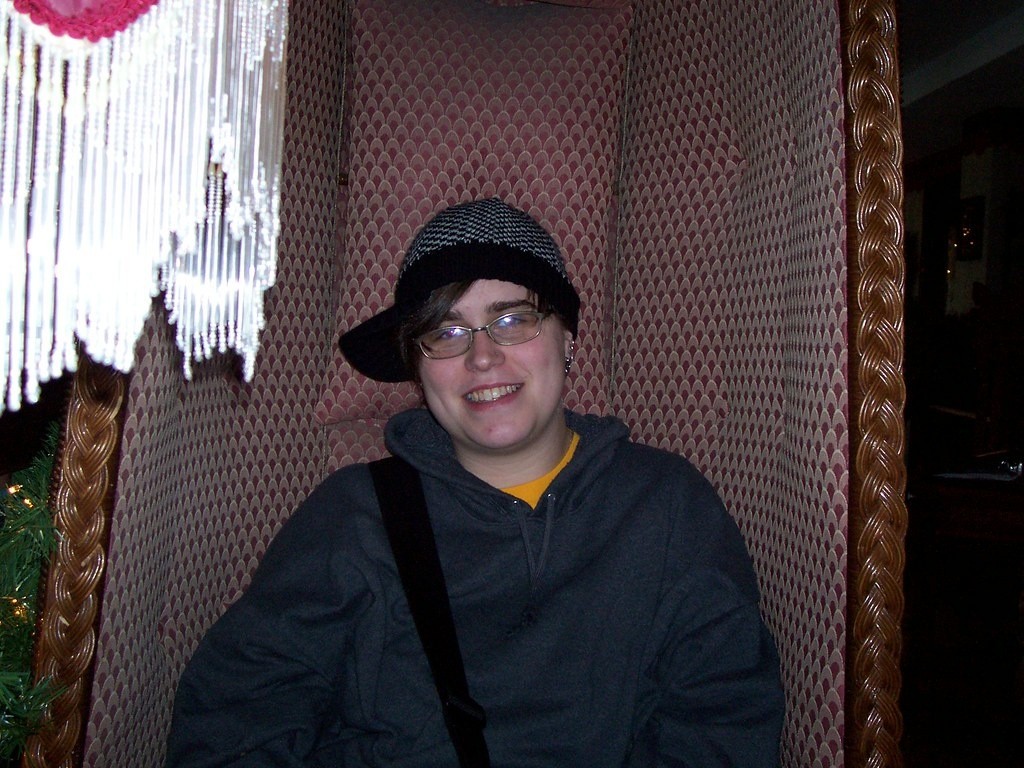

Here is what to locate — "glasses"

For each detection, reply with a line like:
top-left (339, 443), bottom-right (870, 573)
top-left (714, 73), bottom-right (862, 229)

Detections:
top-left (409, 309), bottom-right (556, 359)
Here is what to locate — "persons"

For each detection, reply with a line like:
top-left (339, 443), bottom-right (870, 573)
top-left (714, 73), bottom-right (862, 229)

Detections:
top-left (166, 196), bottom-right (787, 766)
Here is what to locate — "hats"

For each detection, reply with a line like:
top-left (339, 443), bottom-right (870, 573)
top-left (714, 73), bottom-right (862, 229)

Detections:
top-left (337, 197), bottom-right (580, 383)
